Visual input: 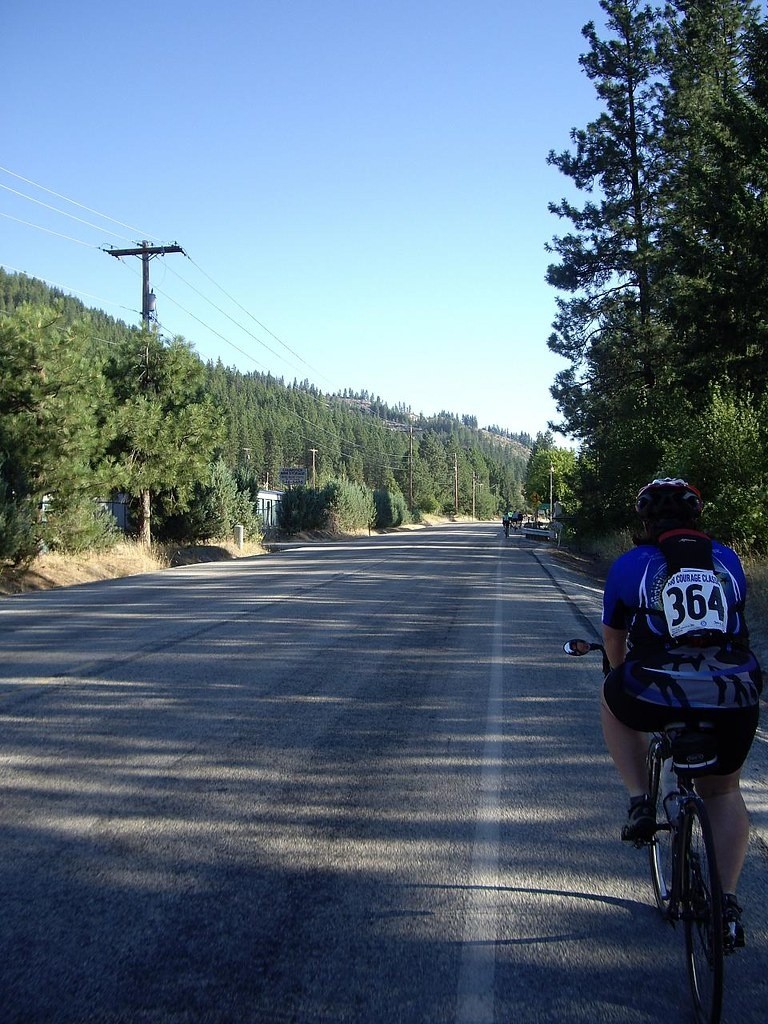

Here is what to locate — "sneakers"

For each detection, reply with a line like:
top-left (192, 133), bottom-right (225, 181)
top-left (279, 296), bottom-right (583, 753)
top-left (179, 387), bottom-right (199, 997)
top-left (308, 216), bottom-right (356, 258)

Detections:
top-left (621, 794), bottom-right (656, 841)
top-left (723, 896), bottom-right (746, 948)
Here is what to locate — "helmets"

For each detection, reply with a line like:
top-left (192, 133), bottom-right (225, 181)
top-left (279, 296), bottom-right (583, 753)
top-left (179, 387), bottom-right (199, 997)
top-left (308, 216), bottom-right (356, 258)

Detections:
top-left (635, 477), bottom-right (702, 517)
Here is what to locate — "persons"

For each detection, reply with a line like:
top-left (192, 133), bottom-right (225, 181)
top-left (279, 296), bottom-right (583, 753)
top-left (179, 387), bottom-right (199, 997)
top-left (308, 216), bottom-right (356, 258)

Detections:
top-left (502, 511), bottom-right (523, 530)
top-left (601, 476), bottom-right (764, 949)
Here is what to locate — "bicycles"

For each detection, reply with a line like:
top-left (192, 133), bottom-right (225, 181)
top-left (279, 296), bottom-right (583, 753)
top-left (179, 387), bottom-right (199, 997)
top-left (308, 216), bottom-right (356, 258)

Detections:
top-left (562, 636), bottom-right (746, 1024)
top-left (503, 520), bottom-right (523, 539)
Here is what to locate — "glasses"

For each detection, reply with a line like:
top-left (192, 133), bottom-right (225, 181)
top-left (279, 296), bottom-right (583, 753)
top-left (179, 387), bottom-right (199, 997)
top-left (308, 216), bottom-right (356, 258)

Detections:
top-left (576, 640), bottom-right (586, 643)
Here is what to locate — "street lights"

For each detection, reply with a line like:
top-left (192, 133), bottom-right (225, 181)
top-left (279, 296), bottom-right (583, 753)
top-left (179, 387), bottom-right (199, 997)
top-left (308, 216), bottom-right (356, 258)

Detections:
top-left (549, 466), bottom-right (563, 546)
top-left (308, 447), bottom-right (319, 500)
top-left (472, 484), bottom-right (484, 523)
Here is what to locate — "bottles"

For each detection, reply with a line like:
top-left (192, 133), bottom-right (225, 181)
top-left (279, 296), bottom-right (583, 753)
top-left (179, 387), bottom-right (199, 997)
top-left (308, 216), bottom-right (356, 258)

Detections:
top-left (659, 757), bottom-right (679, 821)
